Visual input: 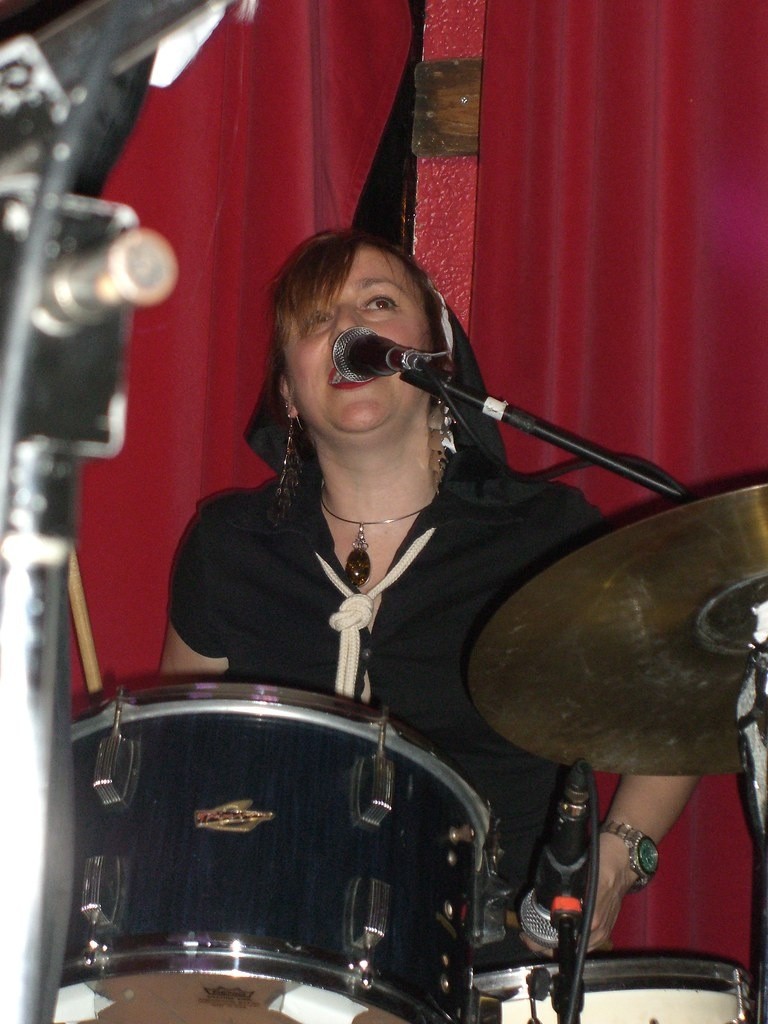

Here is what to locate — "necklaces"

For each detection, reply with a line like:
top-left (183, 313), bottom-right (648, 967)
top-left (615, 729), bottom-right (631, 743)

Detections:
top-left (318, 487), bottom-right (429, 588)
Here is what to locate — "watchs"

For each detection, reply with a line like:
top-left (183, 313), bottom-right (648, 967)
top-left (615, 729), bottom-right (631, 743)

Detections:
top-left (599, 818), bottom-right (659, 896)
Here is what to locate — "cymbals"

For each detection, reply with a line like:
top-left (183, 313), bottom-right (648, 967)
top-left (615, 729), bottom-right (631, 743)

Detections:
top-left (463, 480), bottom-right (768, 775)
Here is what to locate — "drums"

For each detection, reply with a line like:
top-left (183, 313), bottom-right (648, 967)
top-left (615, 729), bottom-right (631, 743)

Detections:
top-left (56, 682), bottom-right (488, 1024)
top-left (472, 954), bottom-right (754, 1022)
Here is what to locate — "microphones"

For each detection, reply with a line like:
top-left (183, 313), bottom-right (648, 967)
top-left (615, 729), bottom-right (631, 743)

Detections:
top-left (330, 327), bottom-right (427, 383)
top-left (521, 757), bottom-right (592, 949)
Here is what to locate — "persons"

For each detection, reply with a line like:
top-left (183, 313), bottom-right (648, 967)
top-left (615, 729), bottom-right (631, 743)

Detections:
top-left (160, 225), bottom-right (694, 960)
